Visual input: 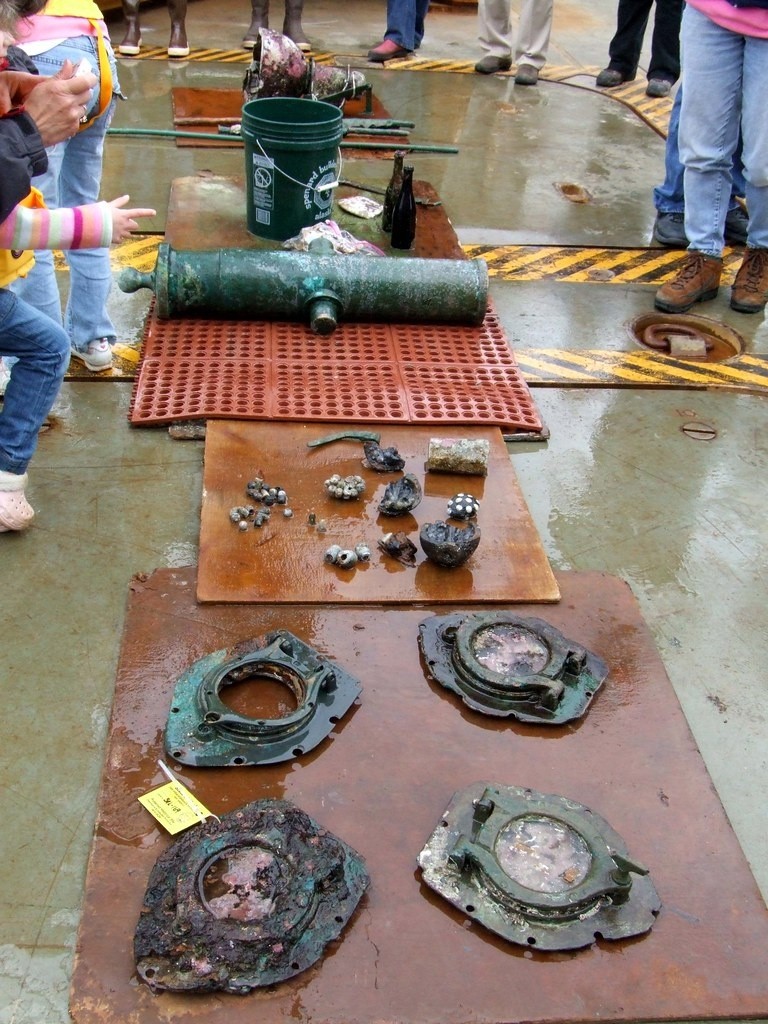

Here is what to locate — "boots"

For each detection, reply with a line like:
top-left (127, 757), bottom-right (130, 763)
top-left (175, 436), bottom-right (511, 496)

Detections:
top-left (241, 0), bottom-right (268, 48)
top-left (283, 0), bottom-right (311, 52)
top-left (119, 0), bottom-right (142, 54)
top-left (167, 0), bottom-right (190, 56)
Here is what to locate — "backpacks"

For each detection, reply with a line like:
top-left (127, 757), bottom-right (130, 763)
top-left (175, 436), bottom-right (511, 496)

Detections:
top-left (0, 186), bottom-right (44, 287)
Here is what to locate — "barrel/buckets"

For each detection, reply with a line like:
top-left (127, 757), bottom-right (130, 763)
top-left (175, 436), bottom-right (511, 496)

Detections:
top-left (241, 97), bottom-right (342, 241)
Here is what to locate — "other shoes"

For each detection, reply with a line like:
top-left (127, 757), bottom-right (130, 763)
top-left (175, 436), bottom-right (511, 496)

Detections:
top-left (514, 63), bottom-right (539, 85)
top-left (597, 68), bottom-right (623, 86)
top-left (474, 55), bottom-right (512, 74)
top-left (0, 469), bottom-right (35, 532)
top-left (70, 337), bottom-right (114, 371)
top-left (647, 79), bottom-right (671, 97)
top-left (368, 39), bottom-right (409, 60)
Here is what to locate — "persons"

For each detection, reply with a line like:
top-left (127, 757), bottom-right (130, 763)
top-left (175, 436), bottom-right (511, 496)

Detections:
top-left (243, 0), bottom-right (312, 52)
top-left (475, 0), bottom-right (553, 85)
top-left (119, 0), bottom-right (189, 56)
top-left (654, 0), bottom-right (768, 314)
top-left (368, 0), bottom-right (428, 60)
top-left (2, 0), bottom-right (127, 372)
top-left (596, 0), bottom-right (686, 97)
top-left (0, 0), bottom-right (156, 532)
top-left (0, 58), bottom-right (98, 225)
top-left (653, 0), bottom-right (760, 246)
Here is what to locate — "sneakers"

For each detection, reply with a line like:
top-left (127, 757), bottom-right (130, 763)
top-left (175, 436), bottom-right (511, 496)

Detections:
top-left (654, 212), bottom-right (690, 246)
top-left (655, 249), bottom-right (723, 314)
top-left (724, 206), bottom-right (749, 247)
top-left (731, 245), bottom-right (768, 314)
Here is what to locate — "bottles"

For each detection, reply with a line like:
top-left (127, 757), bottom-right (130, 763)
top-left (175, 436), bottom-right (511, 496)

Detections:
top-left (391, 167), bottom-right (416, 250)
top-left (383, 151), bottom-right (404, 230)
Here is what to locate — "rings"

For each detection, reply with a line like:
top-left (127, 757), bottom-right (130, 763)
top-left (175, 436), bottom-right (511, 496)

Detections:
top-left (83, 105), bottom-right (87, 110)
